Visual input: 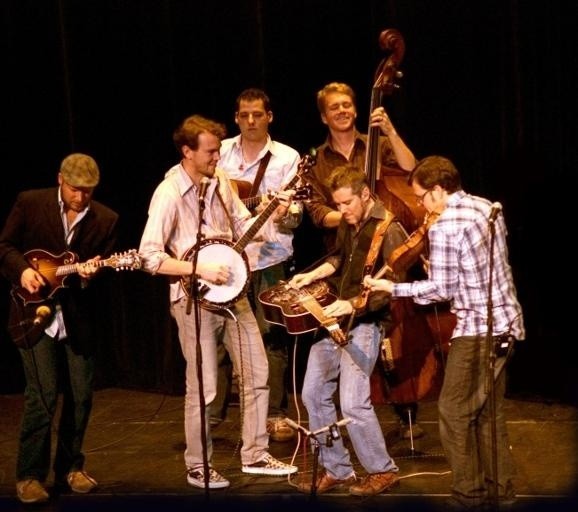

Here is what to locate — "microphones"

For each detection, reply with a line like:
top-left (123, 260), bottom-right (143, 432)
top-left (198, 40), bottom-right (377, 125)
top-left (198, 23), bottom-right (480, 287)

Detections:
top-left (486, 199), bottom-right (504, 225)
top-left (33, 306), bottom-right (50, 325)
top-left (196, 173), bottom-right (213, 202)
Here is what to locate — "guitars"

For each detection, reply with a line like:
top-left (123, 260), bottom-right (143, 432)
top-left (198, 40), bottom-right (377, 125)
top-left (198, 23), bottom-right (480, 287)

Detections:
top-left (229, 179), bottom-right (315, 218)
top-left (257, 275), bottom-right (354, 350)
top-left (11, 250), bottom-right (143, 307)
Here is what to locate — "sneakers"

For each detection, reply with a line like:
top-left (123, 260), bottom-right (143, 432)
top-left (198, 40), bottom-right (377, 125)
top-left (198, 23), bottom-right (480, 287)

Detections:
top-left (265, 415), bottom-right (295, 441)
top-left (188, 466), bottom-right (231, 489)
top-left (240, 455), bottom-right (298, 475)
top-left (68, 469), bottom-right (99, 494)
top-left (399, 419), bottom-right (422, 440)
top-left (14, 478), bottom-right (48, 502)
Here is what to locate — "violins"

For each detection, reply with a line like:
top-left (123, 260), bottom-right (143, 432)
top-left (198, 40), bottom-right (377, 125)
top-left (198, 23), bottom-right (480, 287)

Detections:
top-left (352, 212), bottom-right (441, 308)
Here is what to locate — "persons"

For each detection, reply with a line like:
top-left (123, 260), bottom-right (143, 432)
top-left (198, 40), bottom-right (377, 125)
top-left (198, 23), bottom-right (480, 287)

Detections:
top-left (3, 152), bottom-right (120, 505)
top-left (300, 83), bottom-right (427, 438)
top-left (140, 116), bottom-right (297, 488)
top-left (288, 166), bottom-right (417, 494)
top-left (211, 89), bottom-right (300, 441)
top-left (362, 157), bottom-right (525, 508)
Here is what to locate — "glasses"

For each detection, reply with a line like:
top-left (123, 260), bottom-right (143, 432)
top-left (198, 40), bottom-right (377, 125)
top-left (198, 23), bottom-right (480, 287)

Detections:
top-left (414, 190), bottom-right (427, 203)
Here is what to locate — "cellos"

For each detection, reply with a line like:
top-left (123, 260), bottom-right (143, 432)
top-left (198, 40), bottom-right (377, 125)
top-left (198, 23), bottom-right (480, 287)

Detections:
top-left (364, 29), bottom-right (447, 406)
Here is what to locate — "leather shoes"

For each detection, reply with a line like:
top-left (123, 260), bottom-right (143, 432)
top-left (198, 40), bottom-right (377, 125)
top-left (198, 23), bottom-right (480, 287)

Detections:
top-left (300, 473), bottom-right (355, 492)
top-left (349, 470), bottom-right (397, 495)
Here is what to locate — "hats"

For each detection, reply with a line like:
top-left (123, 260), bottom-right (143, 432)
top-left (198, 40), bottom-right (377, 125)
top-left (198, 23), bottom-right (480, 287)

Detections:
top-left (61, 153), bottom-right (100, 188)
